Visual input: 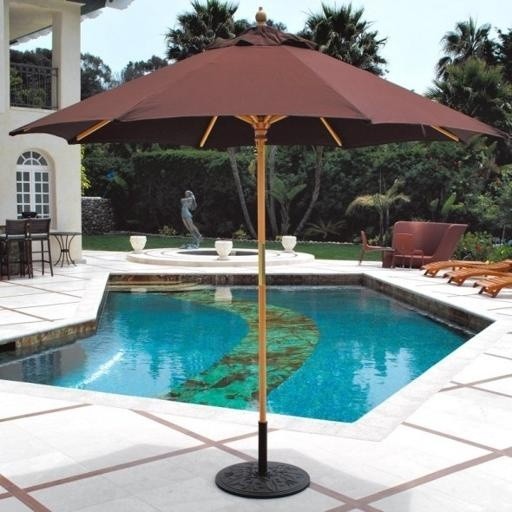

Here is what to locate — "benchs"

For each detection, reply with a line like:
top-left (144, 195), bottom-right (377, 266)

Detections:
top-left (391, 221), bottom-right (467, 267)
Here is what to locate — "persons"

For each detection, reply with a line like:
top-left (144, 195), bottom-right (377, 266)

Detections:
top-left (180, 190), bottom-right (204, 243)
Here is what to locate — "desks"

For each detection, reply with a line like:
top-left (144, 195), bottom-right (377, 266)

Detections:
top-left (50, 232), bottom-right (82, 269)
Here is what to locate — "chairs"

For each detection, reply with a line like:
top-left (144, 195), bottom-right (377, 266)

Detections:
top-left (359, 231), bottom-right (423, 272)
top-left (1, 217), bottom-right (54, 278)
top-left (421, 259), bottom-right (512, 297)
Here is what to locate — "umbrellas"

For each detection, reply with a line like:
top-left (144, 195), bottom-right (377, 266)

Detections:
top-left (7, 7), bottom-right (511, 478)
